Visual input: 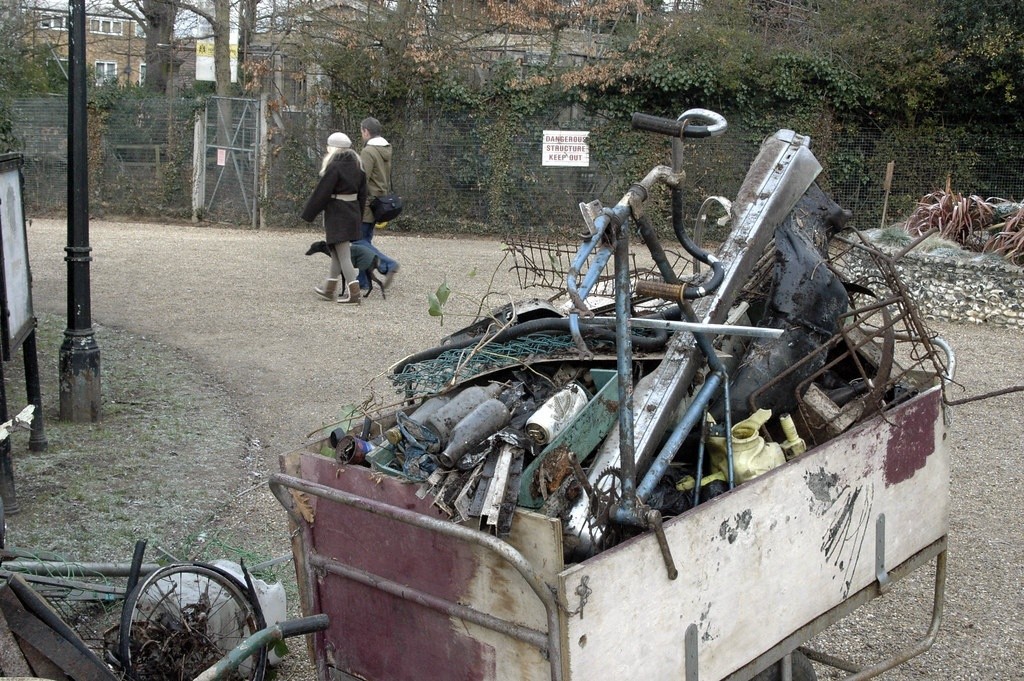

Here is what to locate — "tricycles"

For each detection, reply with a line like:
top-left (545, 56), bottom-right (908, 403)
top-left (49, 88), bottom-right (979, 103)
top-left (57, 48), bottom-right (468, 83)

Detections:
top-left (0, 295), bottom-right (956, 681)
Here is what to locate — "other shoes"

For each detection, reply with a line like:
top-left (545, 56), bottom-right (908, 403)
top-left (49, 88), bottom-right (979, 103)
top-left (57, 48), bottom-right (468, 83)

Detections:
top-left (385, 265), bottom-right (399, 286)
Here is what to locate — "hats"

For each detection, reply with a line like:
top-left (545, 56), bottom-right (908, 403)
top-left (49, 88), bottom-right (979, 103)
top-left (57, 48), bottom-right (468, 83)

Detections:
top-left (327, 133), bottom-right (352, 147)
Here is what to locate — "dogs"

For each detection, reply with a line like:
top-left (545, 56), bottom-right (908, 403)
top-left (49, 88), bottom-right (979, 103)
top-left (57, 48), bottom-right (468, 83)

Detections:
top-left (305, 241), bottom-right (389, 300)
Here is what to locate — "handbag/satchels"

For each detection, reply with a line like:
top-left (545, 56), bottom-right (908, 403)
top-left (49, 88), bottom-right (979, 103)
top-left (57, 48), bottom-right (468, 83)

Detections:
top-left (370, 192), bottom-right (403, 222)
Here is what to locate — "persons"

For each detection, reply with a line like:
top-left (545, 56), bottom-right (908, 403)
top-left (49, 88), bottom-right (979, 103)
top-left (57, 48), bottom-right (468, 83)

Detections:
top-left (353, 117), bottom-right (399, 290)
top-left (300, 132), bottom-right (367, 306)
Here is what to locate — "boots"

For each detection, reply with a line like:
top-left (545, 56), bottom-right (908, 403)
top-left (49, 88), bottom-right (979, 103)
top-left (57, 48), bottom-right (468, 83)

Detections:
top-left (315, 278), bottom-right (338, 300)
top-left (337, 280), bottom-right (361, 305)
